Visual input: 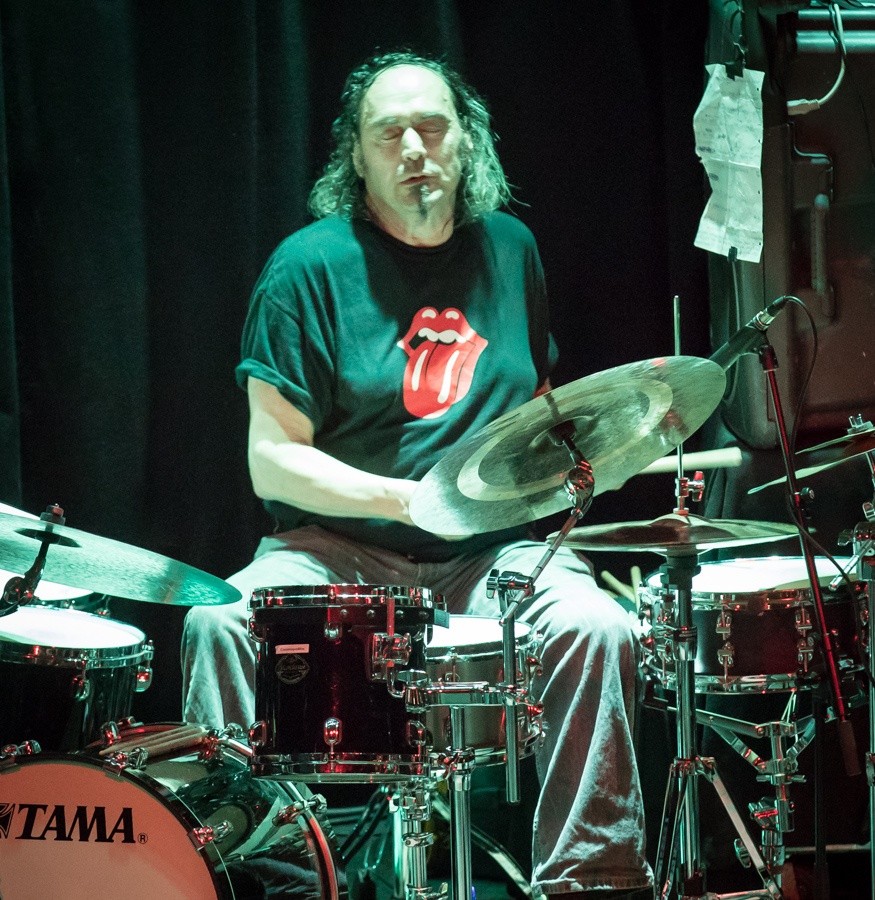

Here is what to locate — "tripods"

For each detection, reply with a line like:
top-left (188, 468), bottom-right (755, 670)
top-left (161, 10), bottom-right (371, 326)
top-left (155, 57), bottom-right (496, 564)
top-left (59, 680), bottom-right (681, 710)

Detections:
top-left (652, 554), bottom-right (783, 900)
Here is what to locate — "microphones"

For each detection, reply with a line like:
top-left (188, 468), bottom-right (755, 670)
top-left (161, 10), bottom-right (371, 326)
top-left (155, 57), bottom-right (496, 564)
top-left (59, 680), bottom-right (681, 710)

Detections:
top-left (709, 296), bottom-right (791, 373)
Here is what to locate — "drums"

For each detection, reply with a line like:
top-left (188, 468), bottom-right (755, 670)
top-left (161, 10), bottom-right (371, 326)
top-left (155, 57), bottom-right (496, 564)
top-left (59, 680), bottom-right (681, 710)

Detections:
top-left (0, 603), bottom-right (147, 759)
top-left (0, 569), bottom-right (112, 618)
top-left (0, 721), bottom-right (341, 900)
top-left (423, 612), bottom-right (546, 770)
top-left (246, 584), bottom-right (438, 784)
top-left (642, 557), bottom-right (869, 692)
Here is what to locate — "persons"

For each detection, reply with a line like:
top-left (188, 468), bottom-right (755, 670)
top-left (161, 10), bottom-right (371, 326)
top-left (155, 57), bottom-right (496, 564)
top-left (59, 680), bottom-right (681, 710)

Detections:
top-left (180, 38), bottom-right (655, 900)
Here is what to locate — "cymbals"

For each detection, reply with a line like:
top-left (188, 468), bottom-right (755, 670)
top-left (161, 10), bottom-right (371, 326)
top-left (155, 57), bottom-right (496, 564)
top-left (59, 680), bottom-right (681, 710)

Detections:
top-left (408, 355), bottom-right (728, 536)
top-left (545, 513), bottom-right (817, 555)
top-left (0, 511), bottom-right (241, 607)
top-left (748, 427), bottom-right (875, 496)
